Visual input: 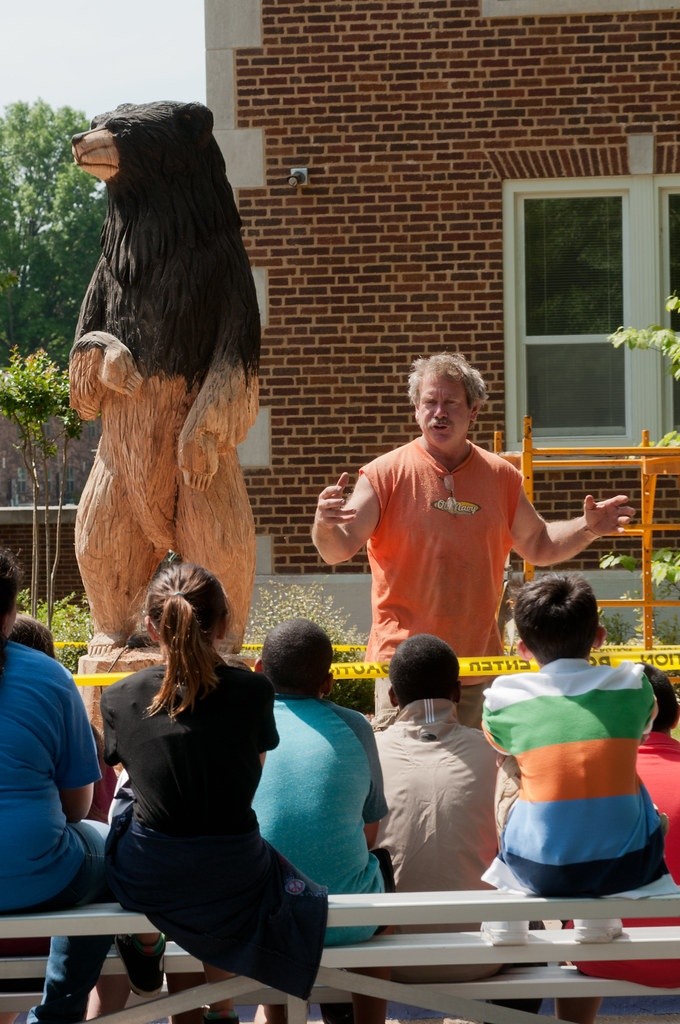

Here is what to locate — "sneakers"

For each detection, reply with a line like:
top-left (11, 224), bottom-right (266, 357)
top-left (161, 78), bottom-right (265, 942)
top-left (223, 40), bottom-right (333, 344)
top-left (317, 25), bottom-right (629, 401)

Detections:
top-left (203, 1010), bottom-right (239, 1024)
top-left (573, 917), bottom-right (623, 943)
top-left (480, 921), bottom-right (529, 946)
top-left (115, 934), bottom-right (166, 997)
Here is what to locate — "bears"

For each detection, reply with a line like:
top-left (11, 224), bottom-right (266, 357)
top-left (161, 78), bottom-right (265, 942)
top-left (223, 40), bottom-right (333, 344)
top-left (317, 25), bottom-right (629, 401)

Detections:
top-left (61, 100), bottom-right (262, 660)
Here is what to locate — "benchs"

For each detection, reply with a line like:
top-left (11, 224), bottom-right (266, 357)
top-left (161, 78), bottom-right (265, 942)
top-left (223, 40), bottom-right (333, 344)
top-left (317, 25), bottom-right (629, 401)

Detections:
top-left (0, 890), bottom-right (680, 1024)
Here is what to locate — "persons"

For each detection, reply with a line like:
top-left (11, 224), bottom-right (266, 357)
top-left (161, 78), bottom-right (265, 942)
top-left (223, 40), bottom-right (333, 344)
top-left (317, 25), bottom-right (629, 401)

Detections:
top-left (553, 662), bottom-right (680, 1024)
top-left (251, 617), bottom-right (396, 1024)
top-left (100, 561), bottom-right (329, 1024)
top-left (311, 353), bottom-right (636, 731)
top-left (374, 632), bottom-right (548, 1015)
top-left (480, 572), bottom-right (680, 946)
top-left (0, 549), bottom-right (118, 1024)
